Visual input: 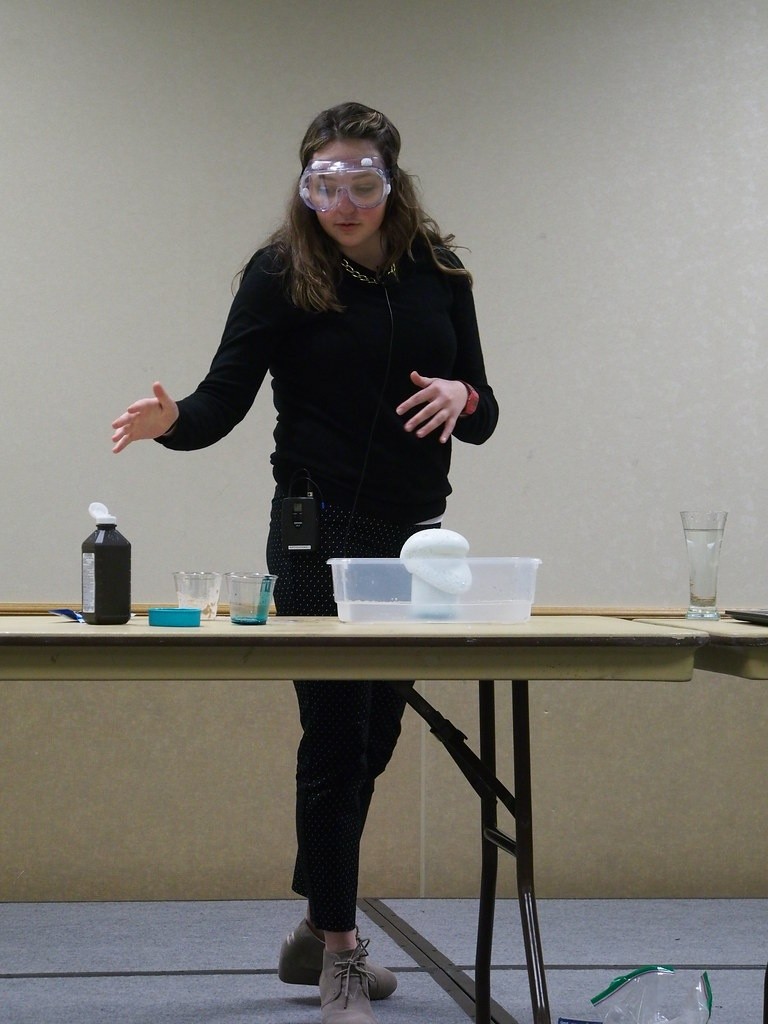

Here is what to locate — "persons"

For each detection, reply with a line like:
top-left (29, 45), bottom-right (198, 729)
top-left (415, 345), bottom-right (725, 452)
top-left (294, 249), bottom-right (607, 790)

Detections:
top-left (112, 101), bottom-right (499, 1024)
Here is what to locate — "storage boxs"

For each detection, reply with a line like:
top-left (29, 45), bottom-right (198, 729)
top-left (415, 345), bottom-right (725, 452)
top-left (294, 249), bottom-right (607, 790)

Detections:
top-left (327, 558), bottom-right (542, 624)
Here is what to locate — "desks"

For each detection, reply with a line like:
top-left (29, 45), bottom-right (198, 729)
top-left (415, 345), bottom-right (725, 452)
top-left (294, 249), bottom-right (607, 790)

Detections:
top-left (0, 615), bottom-right (710, 1024)
top-left (631, 617), bottom-right (768, 680)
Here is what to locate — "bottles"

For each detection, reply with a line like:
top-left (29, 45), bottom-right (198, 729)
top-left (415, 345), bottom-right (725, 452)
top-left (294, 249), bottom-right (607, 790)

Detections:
top-left (81, 501), bottom-right (131, 625)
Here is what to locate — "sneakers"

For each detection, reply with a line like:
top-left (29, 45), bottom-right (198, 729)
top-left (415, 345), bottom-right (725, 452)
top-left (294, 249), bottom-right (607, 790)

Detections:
top-left (319, 937), bottom-right (379, 1024)
top-left (278, 916), bottom-right (397, 1000)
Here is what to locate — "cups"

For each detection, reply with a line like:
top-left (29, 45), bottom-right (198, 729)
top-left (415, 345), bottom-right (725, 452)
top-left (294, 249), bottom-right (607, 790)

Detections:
top-left (225, 572), bottom-right (277, 624)
top-left (681, 511), bottom-right (728, 621)
top-left (172, 571), bottom-right (221, 620)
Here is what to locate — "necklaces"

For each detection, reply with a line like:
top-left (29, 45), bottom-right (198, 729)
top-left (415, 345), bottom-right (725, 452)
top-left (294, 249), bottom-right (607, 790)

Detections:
top-left (340, 255), bottom-right (396, 286)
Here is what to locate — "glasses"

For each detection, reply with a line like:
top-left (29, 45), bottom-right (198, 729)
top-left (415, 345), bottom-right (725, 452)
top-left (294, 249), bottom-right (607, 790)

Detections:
top-left (298, 155), bottom-right (393, 212)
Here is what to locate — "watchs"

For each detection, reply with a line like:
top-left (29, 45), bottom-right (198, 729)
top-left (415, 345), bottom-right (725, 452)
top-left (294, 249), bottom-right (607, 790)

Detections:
top-left (459, 381), bottom-right (479, 415)
